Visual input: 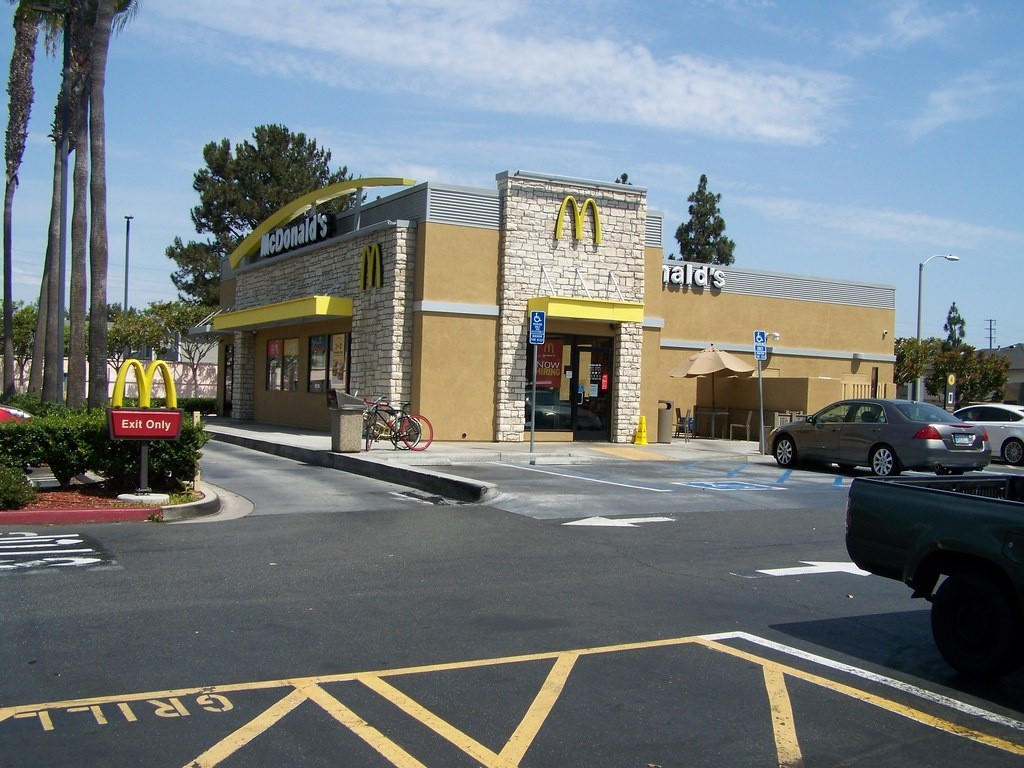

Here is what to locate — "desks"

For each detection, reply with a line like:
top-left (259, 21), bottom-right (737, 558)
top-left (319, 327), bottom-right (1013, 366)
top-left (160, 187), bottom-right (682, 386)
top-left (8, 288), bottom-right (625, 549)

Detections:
top-left (695, 412), bottom-right (730, 439)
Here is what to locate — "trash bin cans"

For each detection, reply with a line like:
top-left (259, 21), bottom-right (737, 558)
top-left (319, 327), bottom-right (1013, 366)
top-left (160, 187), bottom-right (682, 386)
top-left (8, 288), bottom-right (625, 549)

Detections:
top-left (657, 399), bottom-right (674, 444)
top-left (325, 388), bottom-right (368, 453)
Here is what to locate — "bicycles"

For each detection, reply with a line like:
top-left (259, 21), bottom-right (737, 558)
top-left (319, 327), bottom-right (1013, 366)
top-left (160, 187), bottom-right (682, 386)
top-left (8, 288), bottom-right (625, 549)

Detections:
top-left (363, 396), bottom-right (434, 452)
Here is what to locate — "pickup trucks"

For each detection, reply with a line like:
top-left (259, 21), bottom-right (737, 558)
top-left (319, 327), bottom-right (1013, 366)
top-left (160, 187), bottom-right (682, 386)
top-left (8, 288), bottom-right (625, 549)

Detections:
top-left (846, 471), bottom-right (1024, 677)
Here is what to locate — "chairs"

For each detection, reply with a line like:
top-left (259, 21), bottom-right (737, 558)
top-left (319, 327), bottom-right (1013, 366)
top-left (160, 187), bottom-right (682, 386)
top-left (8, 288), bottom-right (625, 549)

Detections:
top-left (672, 409), bottom-right (691, 443)
top-left (861, 411), bottom-right (873, 422)
top-left (730, 411), bottom-right (753, 442)
top-left (675, 408), bottom-right (696, 438)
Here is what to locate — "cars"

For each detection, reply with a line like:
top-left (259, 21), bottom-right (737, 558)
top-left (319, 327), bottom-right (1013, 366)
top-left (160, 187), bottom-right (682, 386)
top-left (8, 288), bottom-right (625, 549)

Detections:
top-left (767, 398), bottom-right (993, 476)
top-left (952, 403), bottom-right (1024, 466)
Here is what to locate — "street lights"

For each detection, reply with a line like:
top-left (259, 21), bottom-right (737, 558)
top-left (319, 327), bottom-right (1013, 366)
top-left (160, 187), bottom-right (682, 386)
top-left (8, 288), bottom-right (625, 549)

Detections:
top-left (757, 332), bottom-right (780, 456)
top-left (913, 252), bottom-right (959, 415)
top-left (124, 215), bottom-right (135, 312)
